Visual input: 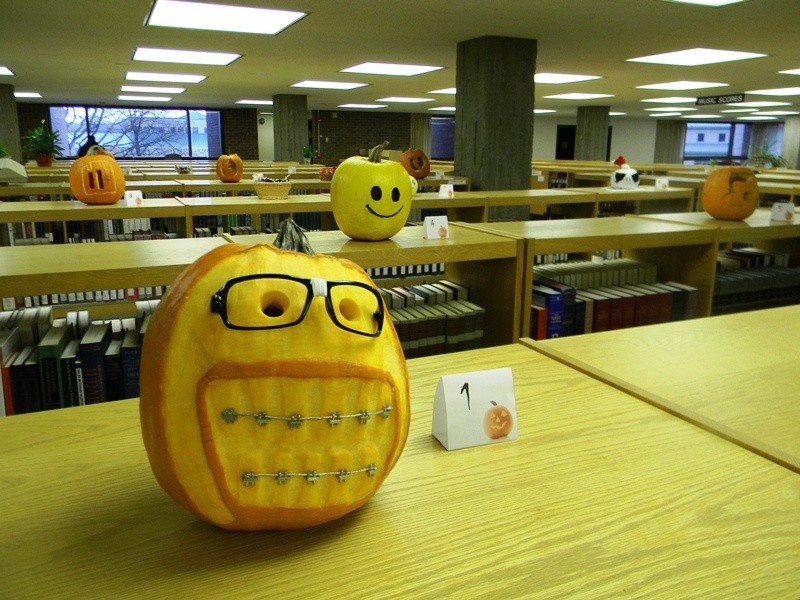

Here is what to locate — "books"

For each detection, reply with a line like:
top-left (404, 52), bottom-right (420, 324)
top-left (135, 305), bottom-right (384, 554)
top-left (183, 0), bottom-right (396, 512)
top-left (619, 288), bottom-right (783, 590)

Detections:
top-left (1, 184), bottom-right (800, 420)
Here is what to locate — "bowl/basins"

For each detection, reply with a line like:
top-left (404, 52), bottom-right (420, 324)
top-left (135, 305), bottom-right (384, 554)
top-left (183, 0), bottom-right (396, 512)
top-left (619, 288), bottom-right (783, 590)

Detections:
top-left (175, 164), bottom-right (192, 175)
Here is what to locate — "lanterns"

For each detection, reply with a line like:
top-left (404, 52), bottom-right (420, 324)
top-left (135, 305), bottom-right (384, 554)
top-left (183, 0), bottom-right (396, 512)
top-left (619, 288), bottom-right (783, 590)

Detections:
top-left (399, 147), bottom-right (429, 180)
top-left (69, 145), bottom-right (124, 206)
top-left (216, 145), bottom-right (242, 182)
top-left (609, 162), bottom-right (639, 188)
top-left (702, 164), bottom-right (758, 221)
top-left (330, 140), bottom-right (410, 243)
top-left (139, 216), bottom-right (411, 532)
top-left (481, 400), bottom-right (512, 439)
top-left (438, 225), bottom-right (447, 238)
